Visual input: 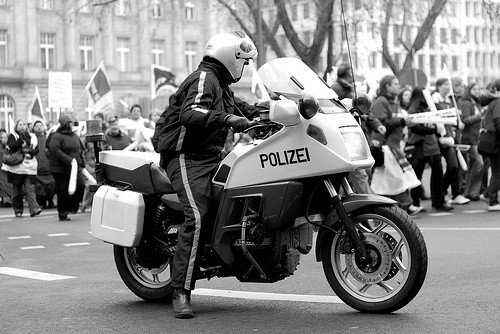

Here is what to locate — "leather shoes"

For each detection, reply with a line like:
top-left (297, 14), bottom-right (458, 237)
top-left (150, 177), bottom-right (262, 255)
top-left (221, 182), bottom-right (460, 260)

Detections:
top-left (171, 288), bottom-right (194, 318)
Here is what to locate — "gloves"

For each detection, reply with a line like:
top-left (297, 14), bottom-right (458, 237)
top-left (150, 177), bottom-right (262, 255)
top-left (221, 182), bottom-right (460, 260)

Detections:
top-left (224, 113), bottom-right (253, 133)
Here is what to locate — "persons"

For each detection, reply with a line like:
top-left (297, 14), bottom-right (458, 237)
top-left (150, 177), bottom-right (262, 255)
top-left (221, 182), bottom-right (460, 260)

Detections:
top-left (0, 105), bottom-right (165, 221)
top-left (332, 63), bottom-right (497, 214)
top-left (151, 31), bottom-right (263, 320)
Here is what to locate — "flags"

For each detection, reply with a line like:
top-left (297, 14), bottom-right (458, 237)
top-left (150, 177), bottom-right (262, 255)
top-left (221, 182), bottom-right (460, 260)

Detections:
top-left (151, 63), bottom-right (182, 103)
top-left (76, 60), bottom-right (115, 122)
top-left (28, 84), bottom-right (47, 125)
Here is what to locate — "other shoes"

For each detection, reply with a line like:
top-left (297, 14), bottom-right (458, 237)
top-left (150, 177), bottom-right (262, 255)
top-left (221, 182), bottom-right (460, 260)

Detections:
top-left (30, 208), bottom-right (41, 217)
top-left (407, 205), bottom-right (424, 215)
top-left (444, 194), bottom-right (453, 203)
top-left (488, 204), bottom-right (500, 211)
top-left (453, 195), bottom-right (471, 205)
top-left (435, 203), bottom-right (454, 211)
top-left (60, 217), bottom-right (70, 221)
top-left (480, 194), bottom-right (491, 203)
top-left (16, 213), bottom-right (22, 217)
top-left (465, 194), bottom-right (480, 201)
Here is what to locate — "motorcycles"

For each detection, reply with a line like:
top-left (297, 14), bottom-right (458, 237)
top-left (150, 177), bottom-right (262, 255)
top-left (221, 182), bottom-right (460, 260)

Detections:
top-left (85, 57), bottom-right (428, 315)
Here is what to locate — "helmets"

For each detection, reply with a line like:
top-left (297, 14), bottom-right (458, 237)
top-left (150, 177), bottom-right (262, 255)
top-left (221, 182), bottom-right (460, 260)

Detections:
top-left (203, 33), bottom-right (258, 81)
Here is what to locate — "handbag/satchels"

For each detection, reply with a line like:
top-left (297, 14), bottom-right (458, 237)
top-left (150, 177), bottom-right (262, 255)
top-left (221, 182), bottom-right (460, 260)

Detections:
top-left (2, 149), bottom-right (24, 165)
top-left (68, 158), bottom-right (78, 195)
top-left (81, 168), bottom-right (97, 185)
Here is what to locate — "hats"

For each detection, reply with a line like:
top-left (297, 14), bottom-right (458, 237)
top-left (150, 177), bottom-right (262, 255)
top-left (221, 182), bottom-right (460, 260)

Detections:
top-left (59, 116), bottom-right (73, 127)
